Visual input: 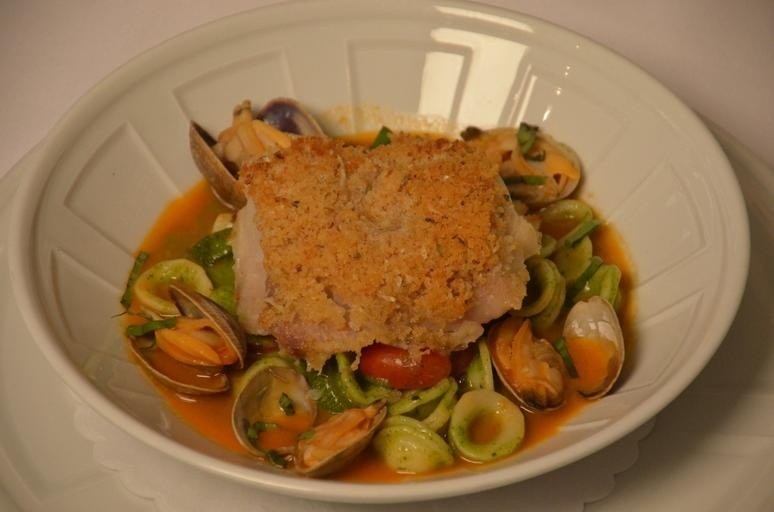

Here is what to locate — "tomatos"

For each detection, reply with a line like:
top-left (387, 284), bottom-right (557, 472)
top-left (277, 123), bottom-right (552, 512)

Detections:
top-left (359, 343), bottom-right (451, 390)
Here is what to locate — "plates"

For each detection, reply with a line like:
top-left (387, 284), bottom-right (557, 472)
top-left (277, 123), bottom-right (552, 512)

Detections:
top-left (10, 2), bottom-right (754, 504)
